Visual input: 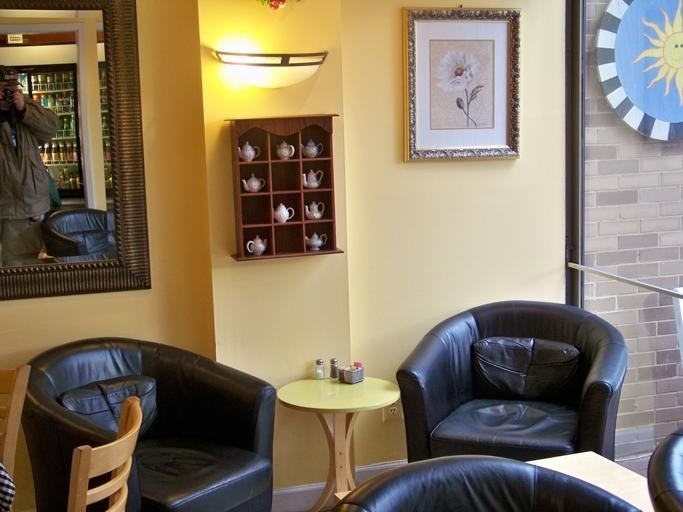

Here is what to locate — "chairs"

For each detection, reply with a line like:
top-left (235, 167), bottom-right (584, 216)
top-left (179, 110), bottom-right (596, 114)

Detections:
top-left (40, 207), bottom-right (116, 256)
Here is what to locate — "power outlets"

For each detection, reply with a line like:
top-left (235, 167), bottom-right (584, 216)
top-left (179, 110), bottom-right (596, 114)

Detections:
top-left (381, 400), bottom-right (402, 422)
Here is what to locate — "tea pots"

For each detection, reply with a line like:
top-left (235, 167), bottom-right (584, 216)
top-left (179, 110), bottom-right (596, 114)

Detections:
top-left (237, 138), bottom-right (325, 253)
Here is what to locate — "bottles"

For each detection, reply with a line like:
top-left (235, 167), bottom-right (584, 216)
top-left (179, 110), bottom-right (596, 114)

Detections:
top-left (329, 357), bottom-right (340, 378)
top-left (316, 359), bottom-right (325, 378)
top-left (26, 69), bottom-right (113, 190)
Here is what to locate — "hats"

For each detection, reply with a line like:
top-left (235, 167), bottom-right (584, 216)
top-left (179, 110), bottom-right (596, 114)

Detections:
top-left (1, 65), bottom-right (24, 87)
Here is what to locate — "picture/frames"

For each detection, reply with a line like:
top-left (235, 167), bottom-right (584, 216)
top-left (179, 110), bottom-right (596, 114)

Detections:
top-left (401, 8), bottom-right (522, 159)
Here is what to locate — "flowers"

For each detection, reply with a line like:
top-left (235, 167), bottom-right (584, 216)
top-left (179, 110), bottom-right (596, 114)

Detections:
top-left (434, 47), bottom-right (484, 129)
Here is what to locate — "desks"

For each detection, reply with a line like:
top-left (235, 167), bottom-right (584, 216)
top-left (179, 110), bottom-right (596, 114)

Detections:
top-left (275, 377), bottom-right (401, 512)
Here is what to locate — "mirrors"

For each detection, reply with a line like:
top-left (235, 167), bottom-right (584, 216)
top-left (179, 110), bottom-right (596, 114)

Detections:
top-left (1, 0), bottom-right (150, 300)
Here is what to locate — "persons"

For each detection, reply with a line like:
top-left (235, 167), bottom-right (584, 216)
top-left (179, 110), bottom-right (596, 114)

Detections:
top-left (0, 64), bottom-right (60, 264)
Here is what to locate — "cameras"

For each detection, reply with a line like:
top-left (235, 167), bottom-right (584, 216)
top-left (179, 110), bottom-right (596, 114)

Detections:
top-left (0, 88), bottom-right (16, 103)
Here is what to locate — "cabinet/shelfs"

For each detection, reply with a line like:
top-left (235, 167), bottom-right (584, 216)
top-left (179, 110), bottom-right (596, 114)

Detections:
top-left (224, 113), bottom-right (343, 260)
top-left (14, 61), bottom-right (114, 200)
